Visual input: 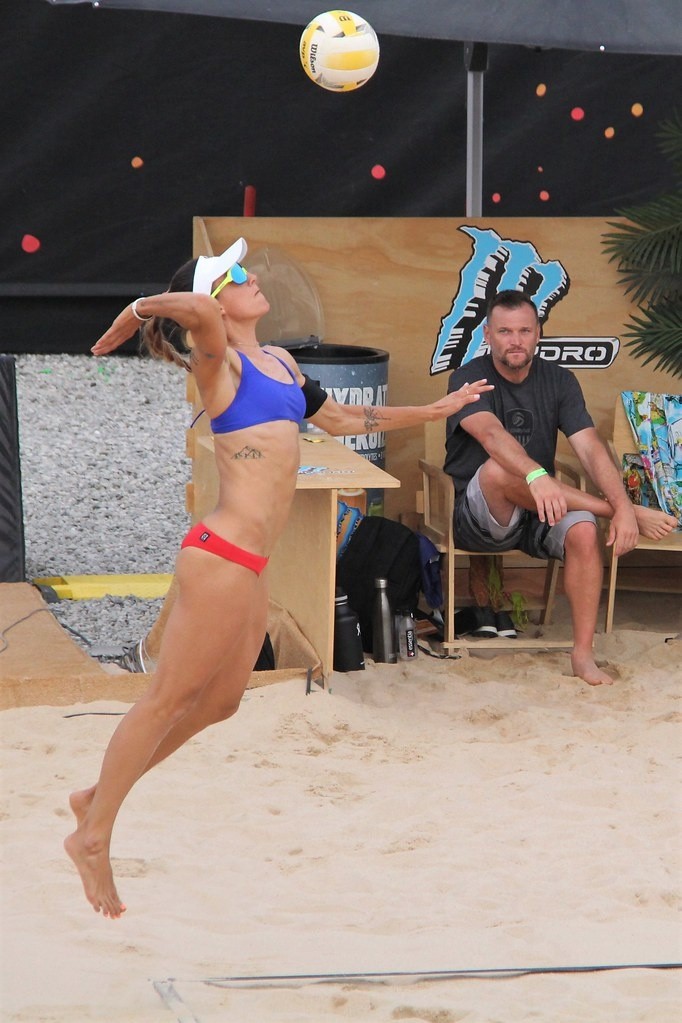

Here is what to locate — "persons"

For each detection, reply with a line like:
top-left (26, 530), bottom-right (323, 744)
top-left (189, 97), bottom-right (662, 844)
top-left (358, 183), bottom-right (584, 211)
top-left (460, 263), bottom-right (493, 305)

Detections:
top-left (64, 238), bottom-right (496, 919)
top-left (442, 290), bottom-right (677, 687)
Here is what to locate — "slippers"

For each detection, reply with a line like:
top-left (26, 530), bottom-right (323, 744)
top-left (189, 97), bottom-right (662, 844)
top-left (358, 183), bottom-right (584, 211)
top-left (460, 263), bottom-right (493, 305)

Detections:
top-left (450, 606), bottom-right (483, 638)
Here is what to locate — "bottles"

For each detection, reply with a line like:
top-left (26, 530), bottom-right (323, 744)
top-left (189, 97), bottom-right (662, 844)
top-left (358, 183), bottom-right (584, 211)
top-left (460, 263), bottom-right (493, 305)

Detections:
top-left (335, 594), bottom-right (364, 671)
top-left (399, 610), bottom-right (418, 660)
top-left (372, 577), bottom-right (397, 663)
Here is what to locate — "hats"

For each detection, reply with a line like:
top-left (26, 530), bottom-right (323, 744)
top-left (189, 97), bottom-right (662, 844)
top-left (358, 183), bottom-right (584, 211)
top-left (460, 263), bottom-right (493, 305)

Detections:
top-left (187, 237), bottom-right (247, 349)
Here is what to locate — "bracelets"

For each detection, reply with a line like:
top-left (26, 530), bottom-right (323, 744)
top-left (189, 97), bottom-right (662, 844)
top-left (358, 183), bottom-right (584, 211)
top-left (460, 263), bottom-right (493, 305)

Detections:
top-left (132, 297), bottom-right (154, 321)
top-left (526, 468), bottom-right (548, 486)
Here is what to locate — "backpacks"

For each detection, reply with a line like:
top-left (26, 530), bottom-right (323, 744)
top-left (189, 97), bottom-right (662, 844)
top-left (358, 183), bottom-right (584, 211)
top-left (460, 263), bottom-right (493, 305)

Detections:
top-left (338, 516), bottom-right (433, 658)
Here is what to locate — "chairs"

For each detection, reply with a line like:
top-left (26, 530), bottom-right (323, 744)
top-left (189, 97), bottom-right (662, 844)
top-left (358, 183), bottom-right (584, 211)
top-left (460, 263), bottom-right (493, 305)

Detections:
top-left (417, 389), bottom-right (682, 658)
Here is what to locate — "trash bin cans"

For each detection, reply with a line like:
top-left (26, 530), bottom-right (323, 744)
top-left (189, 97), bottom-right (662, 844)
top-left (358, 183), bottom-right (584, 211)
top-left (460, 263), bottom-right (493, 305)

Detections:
top-left (243, 248), bottom-right (390, 565)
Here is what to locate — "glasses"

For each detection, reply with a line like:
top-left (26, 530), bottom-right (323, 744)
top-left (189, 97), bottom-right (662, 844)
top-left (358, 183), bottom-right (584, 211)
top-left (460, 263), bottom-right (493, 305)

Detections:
top-left (211, 261), bottom-right (248, 297)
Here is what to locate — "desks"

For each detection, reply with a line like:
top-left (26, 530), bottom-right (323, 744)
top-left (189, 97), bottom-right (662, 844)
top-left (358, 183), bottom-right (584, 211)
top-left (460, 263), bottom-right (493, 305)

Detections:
top-left (197, 428), bottom-right (403, 688)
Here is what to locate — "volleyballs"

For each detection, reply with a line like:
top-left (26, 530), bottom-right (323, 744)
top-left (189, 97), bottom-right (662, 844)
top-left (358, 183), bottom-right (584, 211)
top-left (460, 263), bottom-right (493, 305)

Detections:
top-left (299, 10), bottom-right (380, 94)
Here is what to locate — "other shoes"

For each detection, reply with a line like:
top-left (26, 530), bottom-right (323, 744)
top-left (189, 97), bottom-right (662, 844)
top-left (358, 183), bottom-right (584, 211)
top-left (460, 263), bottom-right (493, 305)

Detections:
top-left (496, 612), bottom-right (518, 638)
top-left (476, 607), bottom-right (497, 637)
